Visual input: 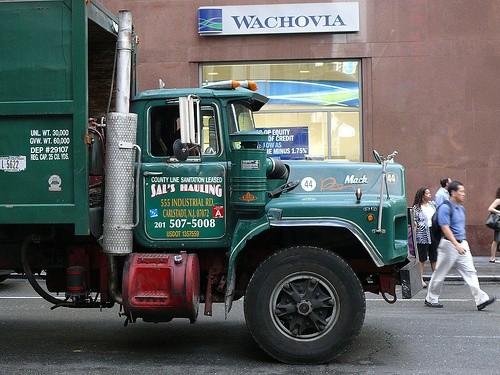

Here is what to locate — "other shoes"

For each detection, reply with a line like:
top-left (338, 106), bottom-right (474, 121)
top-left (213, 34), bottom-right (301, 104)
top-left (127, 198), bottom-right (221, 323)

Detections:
top-left (489, 259), bottom-right (500, 264)
top-left (477, 298), bottom-right (496, 310)
top-left (422, 281), bottom-right (428, 288)
top-left (425, 300), bottom-right (443, 308)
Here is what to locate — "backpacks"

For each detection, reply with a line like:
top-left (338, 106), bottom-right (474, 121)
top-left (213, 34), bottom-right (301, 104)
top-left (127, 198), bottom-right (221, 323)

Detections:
top-left (430, 201), bottom-right (453, 247)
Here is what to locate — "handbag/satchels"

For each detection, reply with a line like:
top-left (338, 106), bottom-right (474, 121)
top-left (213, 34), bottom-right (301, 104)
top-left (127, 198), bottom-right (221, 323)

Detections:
top-left (486, 212), bottom-right (500, 231)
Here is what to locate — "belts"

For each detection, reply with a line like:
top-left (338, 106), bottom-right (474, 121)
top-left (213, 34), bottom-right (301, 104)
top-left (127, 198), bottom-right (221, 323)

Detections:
top-left (444, 236), bottom-right (462, 243)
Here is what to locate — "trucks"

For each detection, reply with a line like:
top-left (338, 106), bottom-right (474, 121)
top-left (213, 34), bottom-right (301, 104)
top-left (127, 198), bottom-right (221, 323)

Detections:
top-left (0, 0), bottom-right (423, 366)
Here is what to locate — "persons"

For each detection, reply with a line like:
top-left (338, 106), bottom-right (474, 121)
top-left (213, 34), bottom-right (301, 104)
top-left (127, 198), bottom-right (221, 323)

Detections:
top-left (424, 180), bottom-right (494, 310)
top-left (485, 187), bottom-right (500, 262)
top-left (434, 177), bottom-right (452, 208)
top-left (412, 186), bottom-right (439, 287)
